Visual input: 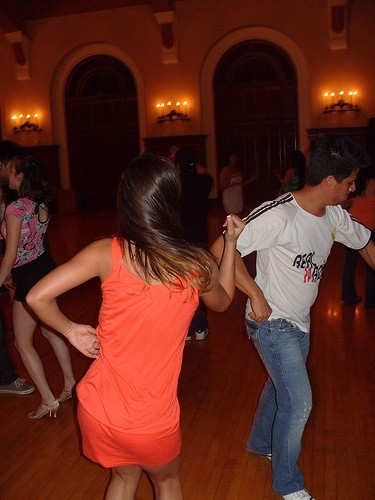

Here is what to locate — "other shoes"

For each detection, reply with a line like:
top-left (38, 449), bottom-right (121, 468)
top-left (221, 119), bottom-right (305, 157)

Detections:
top-left (344, 296), bottom-right (361, 303)
top-left (266, 454), bottom-right (271, 460)
top-left (282, 488), bottom-right (314, 500)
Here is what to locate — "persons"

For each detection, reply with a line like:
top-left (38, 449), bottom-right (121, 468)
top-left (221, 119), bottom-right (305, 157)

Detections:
top-left (164, 141), bottom-right (375, 341)
top-left (209, 135), bottom-right (375, 500)
top-left (0, 139), bottom-right (76, 419)
top-left (26, 156), bottom-right (246, 500)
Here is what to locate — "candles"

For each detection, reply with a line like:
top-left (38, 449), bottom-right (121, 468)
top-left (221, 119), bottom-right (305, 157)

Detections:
top-left (166, 101), bottom-right (171, 112)
top-left (175, 102), bottom-right (180, 112)
top-left (324, 92), bottom-right (328, 105)
top-left (19, 114), bottom-right (23, 125)
top-left (25, 114), bottom-right (30, 122)
top-left (183, 101), bottom-right (187, 115)
top-left (348, 91), bottom-right (357, 105)
top-left (155, 103), bottom-right (164, 116)
top-left (339, 91), bottom-right (344, 100)
top-left (34, 113), bottom-right (38, 126)
top-left (11, 116), bottom-right (16, 126)
top-left (330, 92), bottom-right (335, 105)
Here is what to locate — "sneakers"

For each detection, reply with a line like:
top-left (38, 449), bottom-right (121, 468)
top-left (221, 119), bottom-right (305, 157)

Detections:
top-left (195, 328), bottom-right (210, 340)
top-left (186, 336), bottom-right (192, 340)
top-left (0, 378), bottom-right (36, 394)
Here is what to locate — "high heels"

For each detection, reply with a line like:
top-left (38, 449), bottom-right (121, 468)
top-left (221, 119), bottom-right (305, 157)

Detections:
top-left (26, 400), bottom-right (60, 419)
top-left (55, 392), bottom-right (75, 403)
